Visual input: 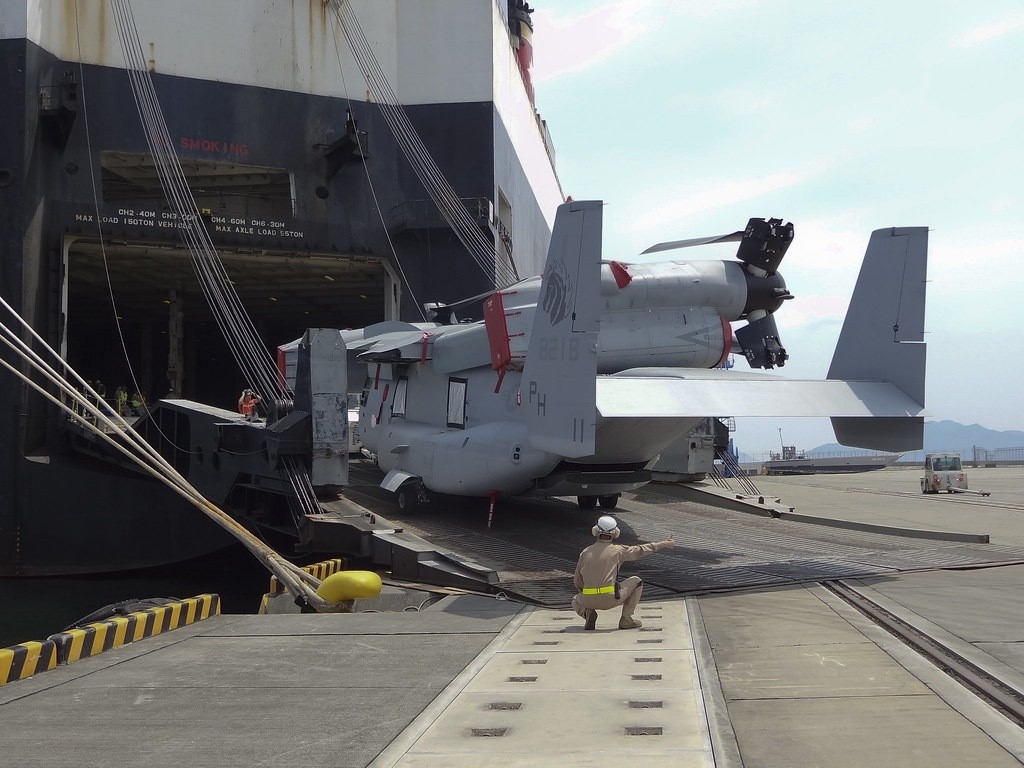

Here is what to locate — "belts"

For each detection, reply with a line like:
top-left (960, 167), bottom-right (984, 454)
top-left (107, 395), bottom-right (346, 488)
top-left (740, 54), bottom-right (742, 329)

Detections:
top-left (583, 586), bottom-right (614, 595)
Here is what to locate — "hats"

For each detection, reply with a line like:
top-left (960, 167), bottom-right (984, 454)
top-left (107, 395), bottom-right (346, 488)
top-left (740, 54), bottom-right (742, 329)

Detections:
top-left (592, 516), bottom-right (620, 538)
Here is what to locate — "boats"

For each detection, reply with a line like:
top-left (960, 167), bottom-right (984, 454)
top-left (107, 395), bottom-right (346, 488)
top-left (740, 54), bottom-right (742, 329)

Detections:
top-left (764, 445), bottom-right (906, 476)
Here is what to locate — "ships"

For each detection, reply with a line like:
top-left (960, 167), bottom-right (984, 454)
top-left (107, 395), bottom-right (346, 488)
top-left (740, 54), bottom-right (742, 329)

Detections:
top-left (0, 2), bottom-right (1023, 650)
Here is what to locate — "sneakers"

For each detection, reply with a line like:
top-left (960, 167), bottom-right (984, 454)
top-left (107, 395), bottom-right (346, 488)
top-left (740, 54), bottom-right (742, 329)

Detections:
top-left (584, 609), bottom-right (597, 630)
top-left (619, 617), bottom-right (642, 629)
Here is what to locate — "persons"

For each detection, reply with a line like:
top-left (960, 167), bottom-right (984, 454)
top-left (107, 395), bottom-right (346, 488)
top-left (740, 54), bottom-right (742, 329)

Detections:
top-left (165, 389), bottom-right (177, 399)
top-left (571, 515), bottom-right (676, 630)
top-left (113, 385), bottom-right (145, 417)
top-left (238, 389), bottom-right (261, 415)
top-left (86, 378), bottom-right (106, 398)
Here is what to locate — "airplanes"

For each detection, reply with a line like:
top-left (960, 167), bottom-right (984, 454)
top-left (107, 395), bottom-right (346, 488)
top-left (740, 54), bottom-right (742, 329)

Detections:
top-left (276, 198), bottom-right (928, 513)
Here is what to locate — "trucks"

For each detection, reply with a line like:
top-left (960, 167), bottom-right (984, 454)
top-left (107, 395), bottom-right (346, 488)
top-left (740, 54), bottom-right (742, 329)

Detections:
top-left (920, 452), bottom-right (991, 496)
